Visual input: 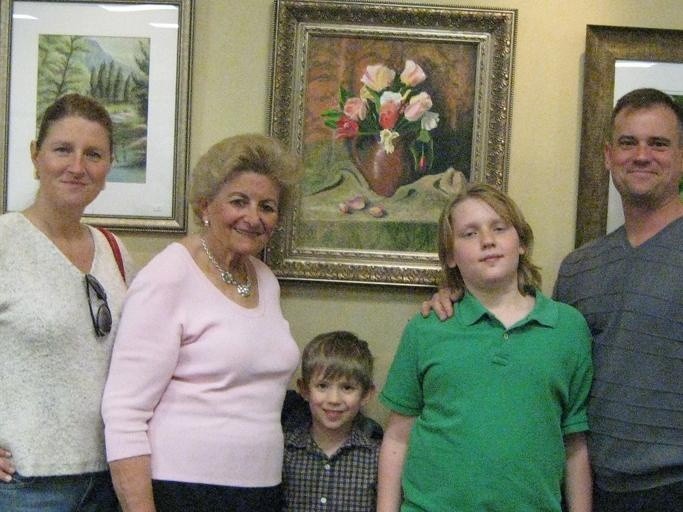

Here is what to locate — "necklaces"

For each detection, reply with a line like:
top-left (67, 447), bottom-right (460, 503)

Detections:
top-left (200, 237), bottom-right (252, 297)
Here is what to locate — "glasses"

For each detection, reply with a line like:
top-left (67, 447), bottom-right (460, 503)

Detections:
top-left (84, 274), bottom-right (111, 338)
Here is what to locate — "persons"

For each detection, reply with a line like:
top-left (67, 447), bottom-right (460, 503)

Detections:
top-left (100, 133), bottom-right (305, 512)
top-left (376, 185), bottom-right (594, 512)
top-left (280, 329), bottom-right (384, 512)
top-left (420, 88), bottom-right (683, 512)
top-left (0, 93), bottom-right (136, 511)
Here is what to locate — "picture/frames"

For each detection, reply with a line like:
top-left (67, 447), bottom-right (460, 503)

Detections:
top-left (261, 0), bottom-right (519, 289)
top-left (0, 0), bottom-right (195, 235)
top-left (574, 23), bottom-right (683, 249)
